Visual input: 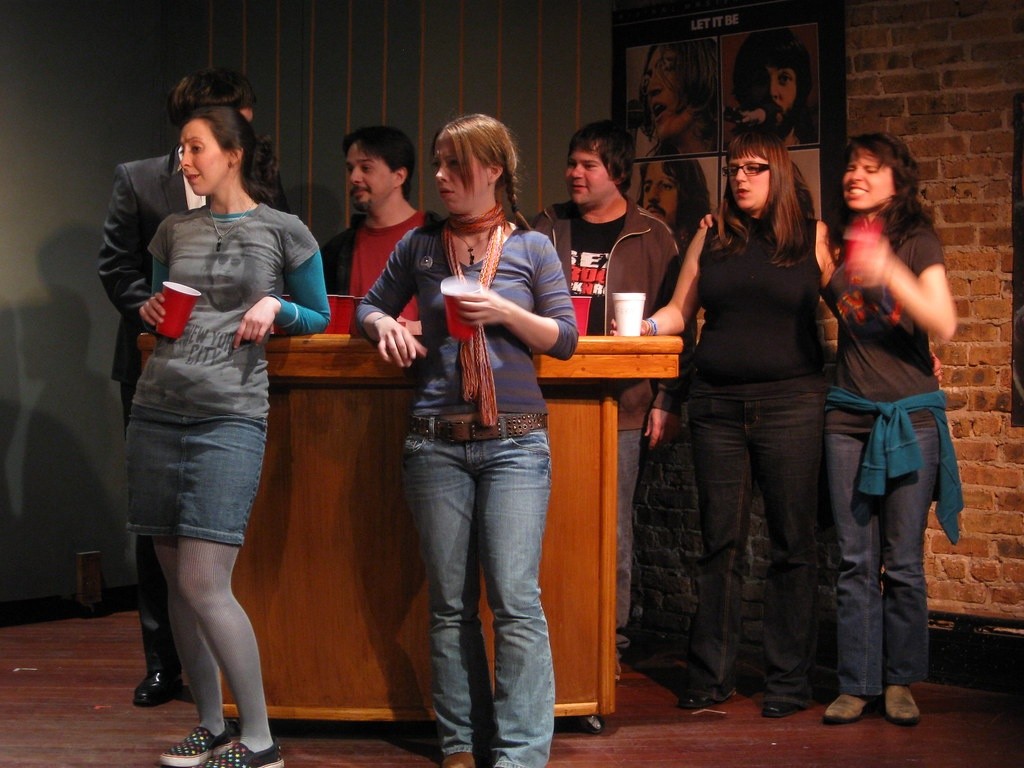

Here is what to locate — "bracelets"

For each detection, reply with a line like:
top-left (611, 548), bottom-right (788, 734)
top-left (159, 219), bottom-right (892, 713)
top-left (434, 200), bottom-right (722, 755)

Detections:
top-left (371, 314), bottom-right (386, 330)
top-left (643, 318), bottom-right (657, 336)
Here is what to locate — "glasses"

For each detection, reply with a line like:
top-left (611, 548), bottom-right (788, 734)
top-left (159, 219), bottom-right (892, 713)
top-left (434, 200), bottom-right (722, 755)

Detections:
top-left (720, 160), bottom-right (773, 178)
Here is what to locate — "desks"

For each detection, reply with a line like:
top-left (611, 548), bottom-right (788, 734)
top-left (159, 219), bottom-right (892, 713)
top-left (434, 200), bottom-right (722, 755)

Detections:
top-left (222, 336), bottom-right (682, 734)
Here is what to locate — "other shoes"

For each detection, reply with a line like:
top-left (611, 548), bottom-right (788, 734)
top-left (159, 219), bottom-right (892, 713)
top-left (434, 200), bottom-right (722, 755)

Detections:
top-left (442, 752), bottom-right (483, 768)
top-left (159, 720), bottom-right (233, 768)
top-left (192, 735), bottom-right (285, 768)
top-left (763, 700), bottom-right (807, 718)
top-left (884, 683), bottom-right (920, 725)
top-left (677, 692), bottom-right (718, 708)
top-left (822, 691), bottom-right (877, 724)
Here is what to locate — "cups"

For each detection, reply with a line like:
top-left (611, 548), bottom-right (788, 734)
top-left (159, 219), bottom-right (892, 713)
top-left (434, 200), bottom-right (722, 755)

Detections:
top-left (273, 294), bottom-right (366, 334)
top-left (570, 297), bottom-right (592, 336)
top-left (844, 233), bottom-right (879, 286)
top-left (156, 281), bottom-right (202, 338)
top-left (612, 292), bottom-right (647, 337)
top-left (441, 275), bottom-right (483, 340)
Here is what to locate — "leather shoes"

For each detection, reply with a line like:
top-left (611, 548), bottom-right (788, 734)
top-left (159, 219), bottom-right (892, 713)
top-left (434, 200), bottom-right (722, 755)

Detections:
top-left (133, 669), bottom-right (183, 705)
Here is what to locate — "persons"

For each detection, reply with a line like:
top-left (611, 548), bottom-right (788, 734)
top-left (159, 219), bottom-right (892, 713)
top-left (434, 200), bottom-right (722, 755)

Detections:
top-left (638, 39), bottom-right (717, 155)
top-left (700, 131), bottom-right (965, 725)
top-left (125, 105), bottom-right (331, 768)
top-left (97, 69), bottom-right (291, 707)
top-left (725, 28), bottom-right (813, 149)
top-left (530, 119), bottom-right (697, 680)
top-left (637, 160), bottom-right (711, 253)
top-left (354, 115), bottom-right (579, 768)
top-left (610, 126), bottom-right (942, 719)
top-left (315, 127), bottom-right (443, 334)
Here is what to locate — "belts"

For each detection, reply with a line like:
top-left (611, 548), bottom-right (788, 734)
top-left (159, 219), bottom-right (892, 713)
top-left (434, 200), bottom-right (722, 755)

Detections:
top-left (405, 409), bottom-right (550, 444)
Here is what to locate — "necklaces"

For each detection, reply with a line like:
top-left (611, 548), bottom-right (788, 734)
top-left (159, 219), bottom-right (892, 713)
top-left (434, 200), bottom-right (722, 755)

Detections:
top-left (457, 234), bottom-right (480, 266)
top-left (208, 198), bottom-right (254, 251)
